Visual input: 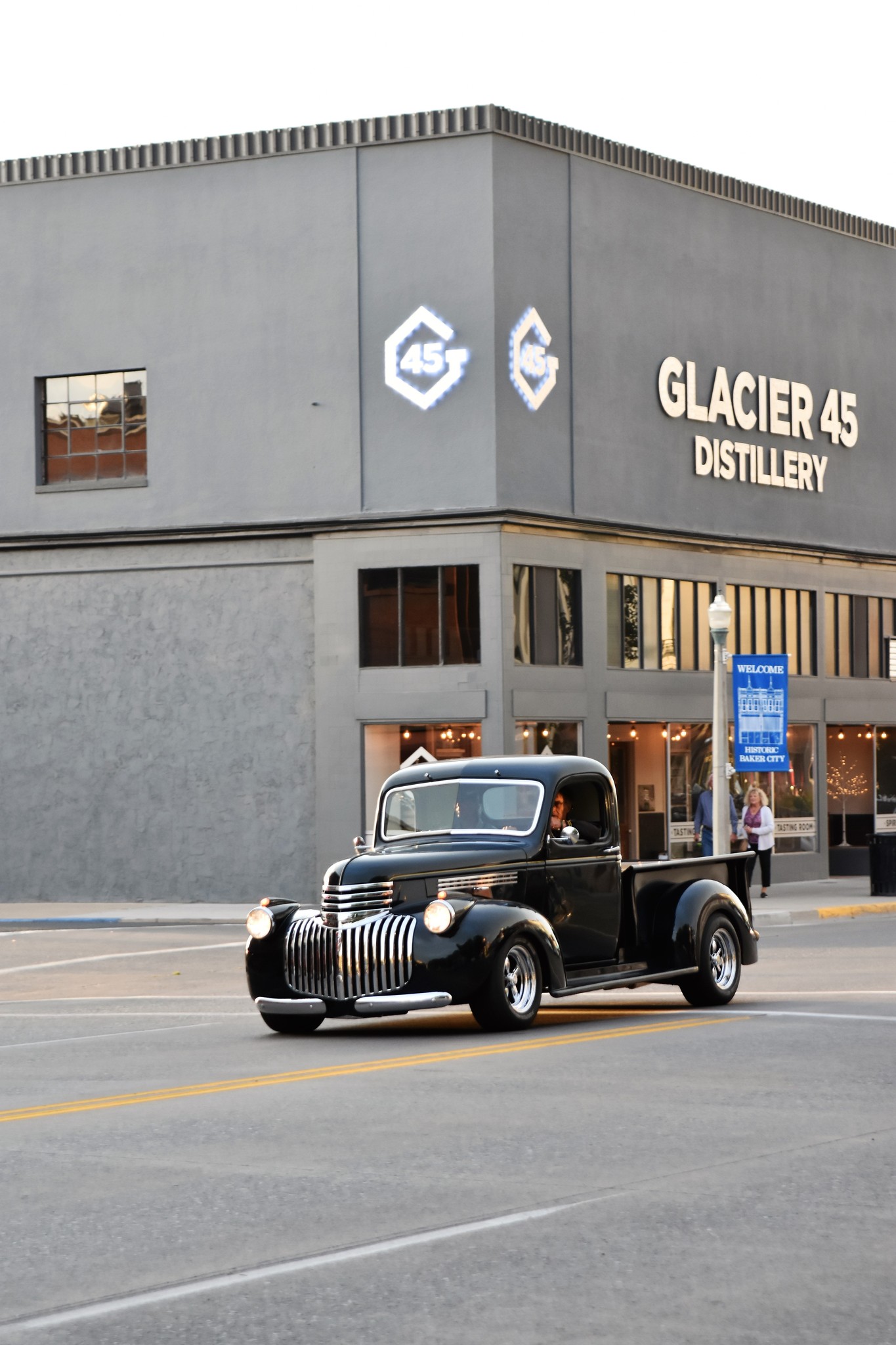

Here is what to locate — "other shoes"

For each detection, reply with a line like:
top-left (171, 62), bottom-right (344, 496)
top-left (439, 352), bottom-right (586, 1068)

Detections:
top-left (760, 892), bottom-right (768, 898)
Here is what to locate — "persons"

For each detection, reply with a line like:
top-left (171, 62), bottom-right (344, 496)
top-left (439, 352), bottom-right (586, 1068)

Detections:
top-left (453, 793), bottom-right (496, 829)
top-left (737, 787), bottom-right (775, 898)
top-left (694, 774), bottom-right (738, 857)
top-left (501, 793), bottom-right (601, 843)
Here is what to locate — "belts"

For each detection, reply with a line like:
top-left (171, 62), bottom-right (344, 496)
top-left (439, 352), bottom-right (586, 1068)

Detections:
top-left (703, 825), bottom-right (712, 832)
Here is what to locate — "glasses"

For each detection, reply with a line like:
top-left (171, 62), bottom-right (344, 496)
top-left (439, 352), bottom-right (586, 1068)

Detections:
top-left (555, 802), bottom-right (564, 808)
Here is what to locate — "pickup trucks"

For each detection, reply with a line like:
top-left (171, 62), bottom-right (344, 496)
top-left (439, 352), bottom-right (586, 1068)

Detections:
top-left (245, 755), bottom-right (759, 1040)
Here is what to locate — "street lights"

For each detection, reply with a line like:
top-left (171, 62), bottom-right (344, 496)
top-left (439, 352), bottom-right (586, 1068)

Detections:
top-left (704, 594), bottom-right (732, 856)
